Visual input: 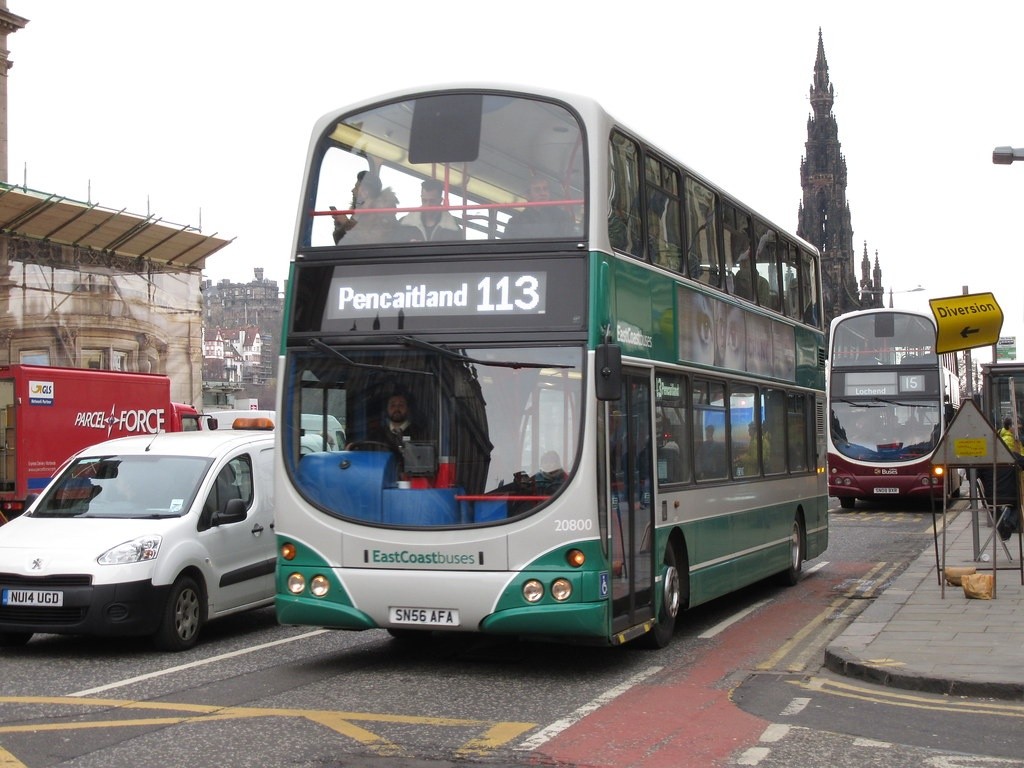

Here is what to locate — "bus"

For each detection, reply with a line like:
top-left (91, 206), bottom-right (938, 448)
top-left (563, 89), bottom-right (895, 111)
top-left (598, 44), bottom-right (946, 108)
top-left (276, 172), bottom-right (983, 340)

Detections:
top-left (277, 85), bottom-right (833, 643)
top-left (827, 308), bottom-right (960, 510)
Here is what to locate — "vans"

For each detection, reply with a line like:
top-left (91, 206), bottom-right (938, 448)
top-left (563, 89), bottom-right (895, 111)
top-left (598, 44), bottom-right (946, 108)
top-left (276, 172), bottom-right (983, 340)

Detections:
top-left (201, 410), bottom-right (347, 452)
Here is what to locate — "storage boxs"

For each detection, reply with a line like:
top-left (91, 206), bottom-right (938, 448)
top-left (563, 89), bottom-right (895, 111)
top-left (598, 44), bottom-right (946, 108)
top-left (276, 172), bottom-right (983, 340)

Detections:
top-left (961, 574), bottom-right (994, 600)
top-left (0, 381), bottom-right (14, 483)
top-left (944, 567), bottom-right (976, 585)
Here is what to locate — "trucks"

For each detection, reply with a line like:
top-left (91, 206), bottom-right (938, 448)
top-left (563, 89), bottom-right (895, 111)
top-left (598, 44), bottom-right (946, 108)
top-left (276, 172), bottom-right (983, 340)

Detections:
top-left (1, 364), bottom-right (218, 517)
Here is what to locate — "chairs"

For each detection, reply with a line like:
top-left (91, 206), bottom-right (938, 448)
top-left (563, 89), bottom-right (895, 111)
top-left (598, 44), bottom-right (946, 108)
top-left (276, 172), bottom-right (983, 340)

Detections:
top-left (623, 223), bottom-right (798, 319)
top-left (834, 353), bottom-right (936, 366)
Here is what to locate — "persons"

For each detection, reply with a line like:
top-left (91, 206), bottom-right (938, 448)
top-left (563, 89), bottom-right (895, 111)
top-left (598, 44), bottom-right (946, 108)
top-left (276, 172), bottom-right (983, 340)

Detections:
top-left (501, 170), bottom-right (575, 239)
top-left (359, 392), bottom-right (434, 473)
top-left (396, 178), bottom-right (463, 241)
top-left (734, 420), bottom-right (772, 476)
top-left (998, 417), bottom-right (1024, 458)
top-left (736, 257), bottom-right (770, 306)
top-left (330, 171), bottom-right (400, 246)
top-left (700, 425), bottom-right (721, 467)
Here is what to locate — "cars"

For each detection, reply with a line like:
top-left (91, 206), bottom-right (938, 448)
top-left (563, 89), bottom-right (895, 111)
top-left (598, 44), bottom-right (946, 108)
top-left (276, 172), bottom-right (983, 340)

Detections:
top-left (0, 429), bottom-right (336, 655)
